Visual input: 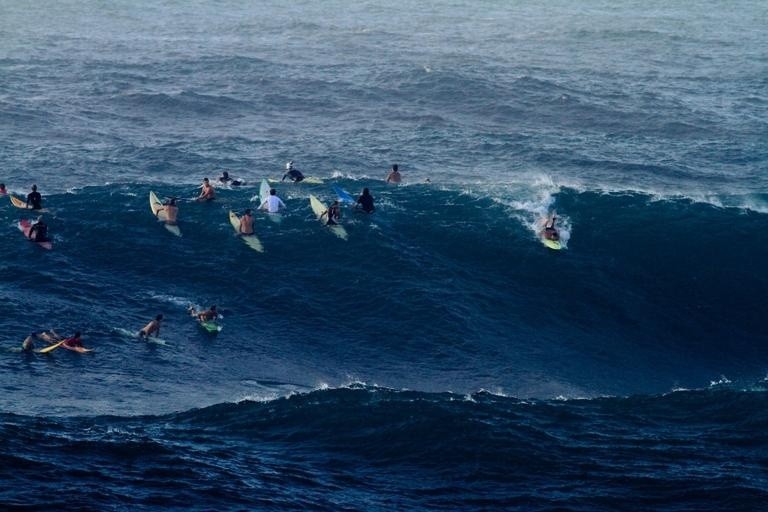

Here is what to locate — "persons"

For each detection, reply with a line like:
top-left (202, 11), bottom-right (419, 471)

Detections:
top-left (279, 162), bottom-right (305, 185)
top-left (155, 197), bottom-right (178, 226)
top-left (256, 188), bottom-right (287, 214)
top-left (540, 215), bottom-right (558, 235)
top-left (0, 336), bottom-right (32, 353)
top-left (196, 177), bottom-right (215, 204)
top-left (351, 187), bottom-right (376, 215)
top-left (386, 164), bottom-right (401, 184)
top-left (233, 208), bottom-right (256, 239)
top-left (321, 200), bottom-right (340, 227)
top-left (136, 314), bottom-right (163, 341)
top-left (0, 184), bottom-right (10, 196)
top-left (24, 183), bottom-right (43, 213)
top-left (218, 171), bottom-right (233, 185)
top-left (189, 305), bottom-right (218, 323)
top-left (27, 215), bottom-right (53, 243)
top-left (40, 328), bottom-right (84, 349)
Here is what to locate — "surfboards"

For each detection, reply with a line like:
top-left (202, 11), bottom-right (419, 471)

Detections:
top-left (542, 238), bottom-right (561, 250)
top-left (309, 194), bottom-right (347, 240)
top-left (10, 195), bottom-right (50, 215)
top-left (267, 177), bottom-right (324, 184)
top-left (329, 181), bottom-right (360, 206)
top-left (6, 338), bottom-right (66, 354)
top-left (149, 190), bottom-right (183, 238)
top-left (20, 219), bottom-right (52, 250)
top-left (201, 319), bottom-right (223, 334)
top-left (114, 326), bottom-right (165, 345)
top-left (259, 178), bottom-right (271, 208)
top-left (37, 334), bottom-right (94, 354)
top-left (228, 210), bottom-right (265, 254)
top-left (205, 179), bottom-right (257, 190)
